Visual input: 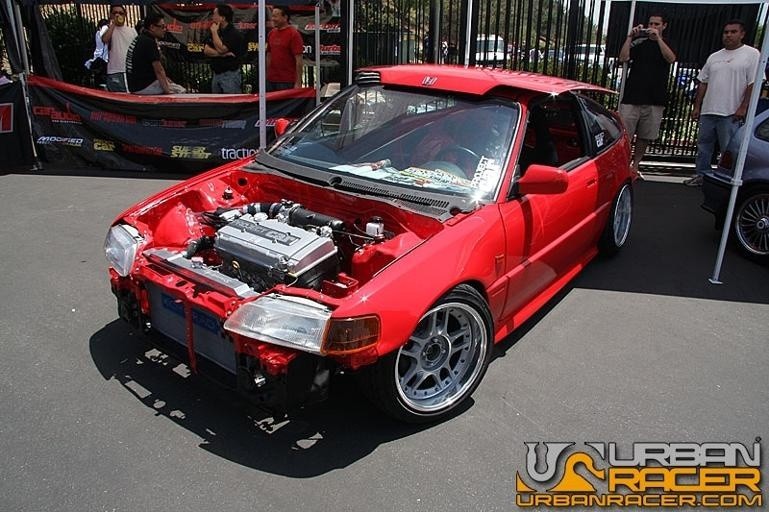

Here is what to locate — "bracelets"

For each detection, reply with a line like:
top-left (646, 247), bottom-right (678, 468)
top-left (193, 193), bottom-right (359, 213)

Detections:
top-left (628, 35), bottom-right (632, 37)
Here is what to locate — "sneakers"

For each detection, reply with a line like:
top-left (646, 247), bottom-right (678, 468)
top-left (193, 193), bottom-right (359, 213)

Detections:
top-left (684, 173), bottom-right (704, 186)
top-left (632, 170), bottom-right (645, 185)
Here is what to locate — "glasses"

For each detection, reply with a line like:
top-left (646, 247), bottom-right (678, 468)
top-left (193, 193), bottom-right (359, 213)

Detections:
top-left (154, 24), bottom-right (166, 30)
top-left (111, 10), bottom-right (125, 15)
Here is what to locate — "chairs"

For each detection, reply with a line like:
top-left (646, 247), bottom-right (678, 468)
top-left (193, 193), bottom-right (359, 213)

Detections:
top-left (456, 101), bottom-right (560, 173)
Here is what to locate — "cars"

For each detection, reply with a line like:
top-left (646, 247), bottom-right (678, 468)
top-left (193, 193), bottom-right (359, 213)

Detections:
top-left (476, 34), bottom-right (618, 73)
top-left (700, 107), bottom-right (769, 263)
top-left (103, 65), bottom-right (640, 424)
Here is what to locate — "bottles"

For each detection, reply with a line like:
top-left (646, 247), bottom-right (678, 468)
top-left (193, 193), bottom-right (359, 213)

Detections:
top-left (117, 15), bottom-right (124, 23)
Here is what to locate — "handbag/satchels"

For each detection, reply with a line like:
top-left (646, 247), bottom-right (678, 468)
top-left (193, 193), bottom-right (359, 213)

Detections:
top-left (90, 56), bottom-right (106, 69)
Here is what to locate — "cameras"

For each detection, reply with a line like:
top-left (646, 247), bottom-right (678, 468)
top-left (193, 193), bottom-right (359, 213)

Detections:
top-left (638, 29), bottom-right (650, 36)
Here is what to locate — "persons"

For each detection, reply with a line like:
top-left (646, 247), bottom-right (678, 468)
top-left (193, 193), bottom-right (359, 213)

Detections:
top-left (126, 20), bottom-right (144, 92)
top-left (102, 4), bottom-right (139, 92)
top-left (267, 6), bottom-right (304, 90)
top-left (132, 14), bottom-right (187, 94)
top-left (204, 5), bottom-right (245, 94)
top-left (529, 48), bottom-right (541, 63)
top-left (424, 32), bottom-right (457, 65)
top-left (684, 19), bottom-right (767, 186)
top-left (618, 14), bottom-right (678, 182)
top-left (84, 19), bottom-right (109, 86)
